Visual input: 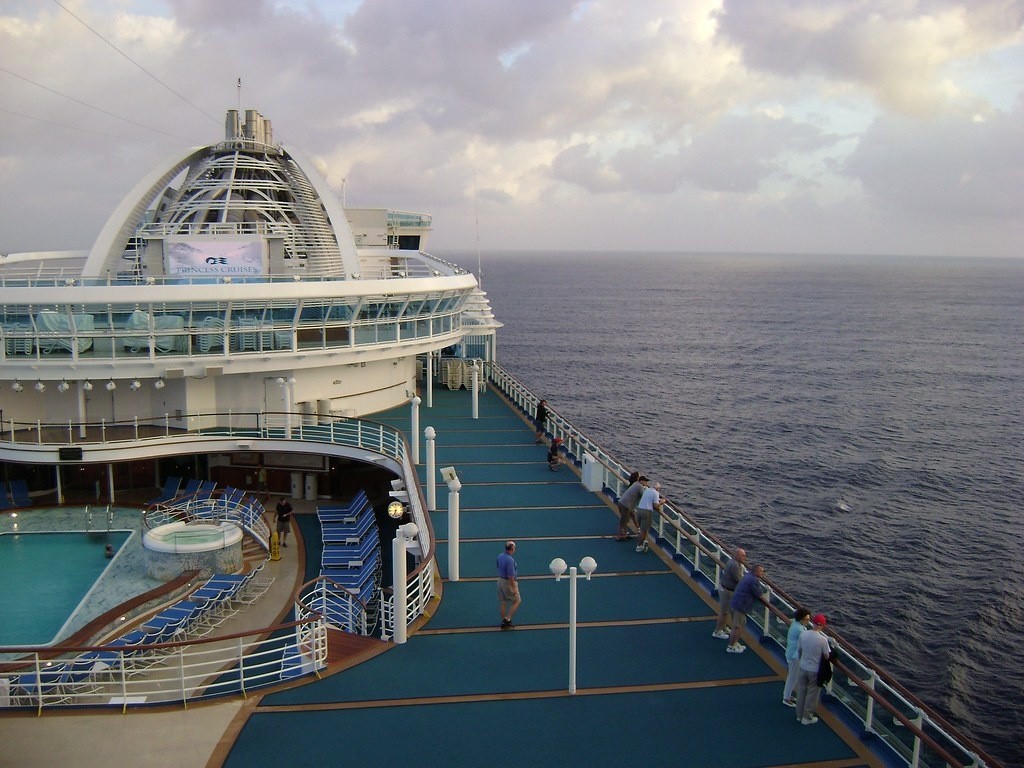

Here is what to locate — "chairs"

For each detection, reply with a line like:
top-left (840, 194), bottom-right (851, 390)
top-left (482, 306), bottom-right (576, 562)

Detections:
top-left (197, 317), bottom-right (294, 353)
top-left (6, 552), bottom-right (278, 705)
top-left (279, 488), bottom-right (382, 681)
top-left (0, 478), bottom-right (35, 509)
top-left (143, 474), bottom-right (265, 528)
top-left (0, 322), bottom-right (33, 355)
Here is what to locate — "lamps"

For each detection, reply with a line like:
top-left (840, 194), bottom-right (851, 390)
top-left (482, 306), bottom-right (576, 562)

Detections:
top-left (33, 379), bottom-right (47, 393)
top-left (64, 277), bottom-right (76, 287)
top-left (154, 378), bottom-right (166, 391)
top-left (222, 275), bottom-right (233, 284)
top-left (350, 272), bottom-right (361, 279)
top-left (433, 269), bottom-right (440, 276)
top-left (145, 275), bottom-right (156, 284)
top-left (291, 274), bottom-right (302, 282)
top-left (83, 380), bottom-right (94, 392)
top-left (130, 379), bottom-right (142, 391)
top-left (398, 270), bottom-right (408, 279)
top-left (57, 379), bottom-right (71, 393)
top-left (10, 378), bottom-right (24, 392)
top-left (106, 379), bottom-right (116, 391)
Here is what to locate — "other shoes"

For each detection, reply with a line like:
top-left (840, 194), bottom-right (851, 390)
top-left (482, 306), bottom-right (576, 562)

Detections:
top-left (279, 542), bottom-right (280, 545)
top-left (535, 439), bottom-right (544, 443)
top-left (549, 465), bottom-right (558, 472)
top-left (643, 540), bottom-right (649, 553)
top-left (501, 618), bottom-right (515, 628)
top-left (782, 698), bottom-right (797, 708)
top-left (790, 696), bottom-right (797, 701)
top-left (636, 544), bottom-right (643, 552)
top-left (283, 543), bottom-right (287, 548)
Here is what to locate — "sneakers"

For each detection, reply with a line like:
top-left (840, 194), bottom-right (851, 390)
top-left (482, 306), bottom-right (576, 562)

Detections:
top-left (801, 716), bottom-right (818, 725)
top-left (712, 629), bottom-right (729, 640)
top-left (723, 625), bottom-right (732, 634)
top-left (797, 718), bottom-right (801, 721)
top-left (726, 642), bottom-right (746, 653)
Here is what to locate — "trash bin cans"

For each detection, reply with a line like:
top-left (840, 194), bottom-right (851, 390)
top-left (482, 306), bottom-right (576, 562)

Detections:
top-left (582, 454), bottom-right (603, 491)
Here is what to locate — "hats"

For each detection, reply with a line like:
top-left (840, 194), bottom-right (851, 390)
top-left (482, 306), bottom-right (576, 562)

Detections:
top-left (639, 476), bottom-right (650, 481)
top-left (814, 614), bottom-right (826, 625)
top-left (555, 438), bottom-right (562, 443)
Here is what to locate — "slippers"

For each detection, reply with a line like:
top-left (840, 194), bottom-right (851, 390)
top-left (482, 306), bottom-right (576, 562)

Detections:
top-left (616, 536), bottom-right (633, 541)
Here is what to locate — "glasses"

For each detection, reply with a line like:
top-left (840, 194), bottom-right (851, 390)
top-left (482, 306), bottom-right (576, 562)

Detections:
top-left (803, 619), bottom-right (810, 621)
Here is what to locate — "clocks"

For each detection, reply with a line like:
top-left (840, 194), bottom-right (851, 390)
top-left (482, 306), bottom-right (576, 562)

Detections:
top-left (387, 502), bottom-right (404, 519)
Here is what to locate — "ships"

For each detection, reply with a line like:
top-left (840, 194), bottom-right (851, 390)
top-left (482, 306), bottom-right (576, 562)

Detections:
top-left (0, 110), bottom-right (998, 768)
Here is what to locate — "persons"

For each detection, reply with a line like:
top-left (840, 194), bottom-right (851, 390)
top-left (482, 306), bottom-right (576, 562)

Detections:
top-left (273, 496), bottom-right (294, 549)
top-left (783, 608), bottom-right (812, 707)
top-left (712, 549), bottom-right (747, 639)
top-left (535, 400), bottom-right (551, 444)
top-left (495, 541), bottom-right (520, 631)
top-left (796, 614), bottom-right (831, 725)
top-left (726, 564), bottom-right (765, 653)
top-left (253, 461), bottom-right (274, 502)
top-left (636, 483), bottom-right (666, 553)
top-left (615, 472), bottom-right (650, 541)
top-left (548, 434), bottom-right (575, 471)
